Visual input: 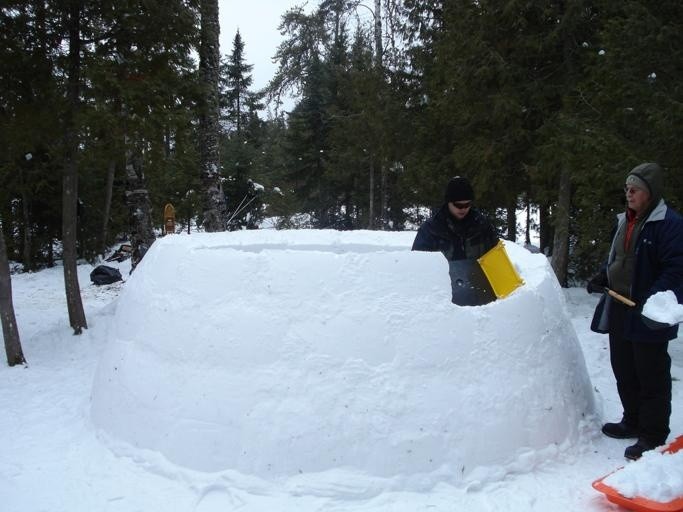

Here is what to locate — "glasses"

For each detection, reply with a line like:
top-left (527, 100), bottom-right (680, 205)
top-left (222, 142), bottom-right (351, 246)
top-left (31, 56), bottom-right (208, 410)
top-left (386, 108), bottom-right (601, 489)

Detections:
top-left (452, 200), bottom-right (472, 209)
top-left (623, 187), bottom-right (641, 194)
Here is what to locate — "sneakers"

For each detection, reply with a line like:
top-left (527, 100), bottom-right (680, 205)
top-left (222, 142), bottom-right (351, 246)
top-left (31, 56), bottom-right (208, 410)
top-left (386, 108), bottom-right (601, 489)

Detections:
top-left (624, 436), bottom-right (658, 460)
top-left (602, 418), bottom-right (640, 439)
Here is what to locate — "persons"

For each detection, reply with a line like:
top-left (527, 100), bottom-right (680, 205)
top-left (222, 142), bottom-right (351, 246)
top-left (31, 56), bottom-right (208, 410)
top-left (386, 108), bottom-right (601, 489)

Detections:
top-left (583, 163), bottom-right (683, 458)
top-left (411, 174), bottom-right (505, 304)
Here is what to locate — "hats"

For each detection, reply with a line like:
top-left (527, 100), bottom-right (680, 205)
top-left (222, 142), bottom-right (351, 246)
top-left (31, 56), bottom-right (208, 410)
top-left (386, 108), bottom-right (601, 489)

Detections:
top-left (446, 178), bottom-right (475, 202)
top-left (625, 174), bottom-right (650, 194)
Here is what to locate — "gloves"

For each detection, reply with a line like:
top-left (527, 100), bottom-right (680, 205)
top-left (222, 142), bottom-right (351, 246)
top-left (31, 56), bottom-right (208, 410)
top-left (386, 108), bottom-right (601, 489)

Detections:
top-left (586, 272), bottom-right (608, 293)
top-left (624, 297), bottom-right (644, 312)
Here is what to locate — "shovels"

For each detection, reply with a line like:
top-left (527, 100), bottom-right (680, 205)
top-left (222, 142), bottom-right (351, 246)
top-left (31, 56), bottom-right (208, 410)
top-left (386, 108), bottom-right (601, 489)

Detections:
top-left (584, 277), bottom-right (683, 329)
